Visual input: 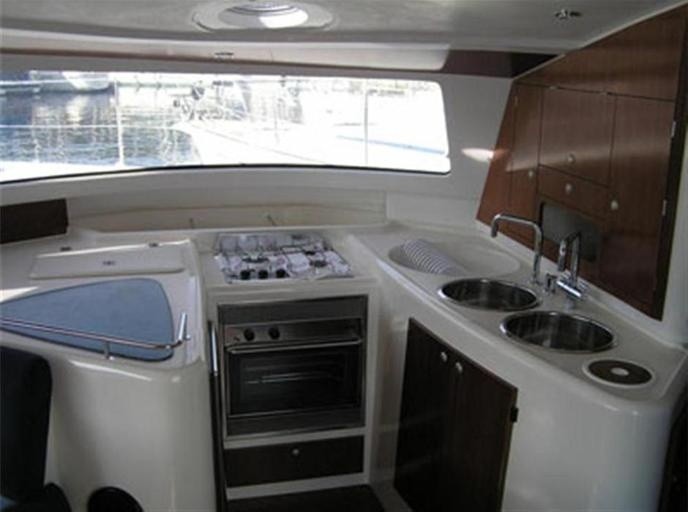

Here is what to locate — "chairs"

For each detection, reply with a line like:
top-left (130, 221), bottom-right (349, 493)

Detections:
top-left (1, 345), bottom-right (54, 512)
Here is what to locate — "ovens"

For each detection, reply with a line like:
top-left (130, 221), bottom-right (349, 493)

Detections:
top-left (218, 298), bottom-right (368, 432)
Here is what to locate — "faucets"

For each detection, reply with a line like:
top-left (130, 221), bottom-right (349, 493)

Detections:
top-left (555, 228), bottom-right (587, 307)
top-left (490, 212), bottom-right (543, 286)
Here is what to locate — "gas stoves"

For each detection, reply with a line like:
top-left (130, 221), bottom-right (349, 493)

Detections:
top-left (216, 228), bottom-right (348, 281)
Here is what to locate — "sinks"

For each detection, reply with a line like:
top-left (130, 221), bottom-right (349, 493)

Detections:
top-left (436, 277), bottom-right (545, 314)
top-left (496, 306), bottom-right (618, 355)
top-left (387, 239), bottom-right (521, 276)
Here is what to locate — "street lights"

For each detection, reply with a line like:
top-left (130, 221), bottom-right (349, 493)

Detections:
top-left (363, 89), bottom-right (378, 165)
